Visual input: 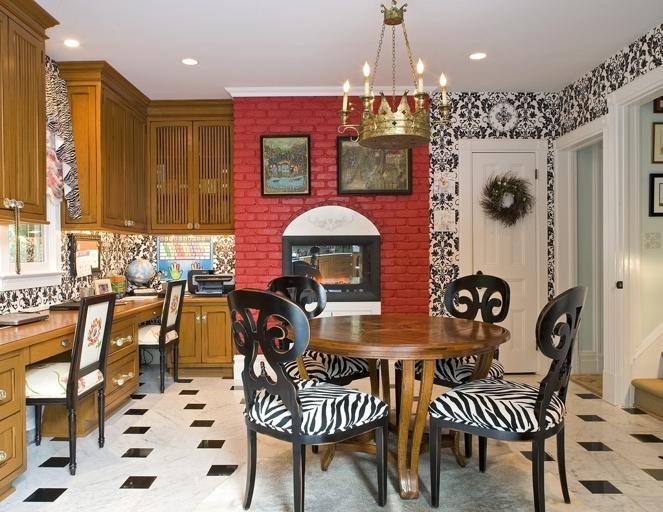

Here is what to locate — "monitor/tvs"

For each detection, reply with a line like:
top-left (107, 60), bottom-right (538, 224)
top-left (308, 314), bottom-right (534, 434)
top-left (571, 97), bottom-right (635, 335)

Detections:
top-left (283, 236), bottom-right (380, 301)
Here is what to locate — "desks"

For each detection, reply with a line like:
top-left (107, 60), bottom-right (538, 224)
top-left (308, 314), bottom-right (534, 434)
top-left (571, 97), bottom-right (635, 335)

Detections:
top-left (0, 293), bottom-right (164, 503)
top-left (278, 312), bottom-right (510, 501)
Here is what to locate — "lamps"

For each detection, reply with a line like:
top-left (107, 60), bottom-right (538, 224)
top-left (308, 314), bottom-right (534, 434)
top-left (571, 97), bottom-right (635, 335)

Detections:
top-left (337, 0), bottom-right (451, 151)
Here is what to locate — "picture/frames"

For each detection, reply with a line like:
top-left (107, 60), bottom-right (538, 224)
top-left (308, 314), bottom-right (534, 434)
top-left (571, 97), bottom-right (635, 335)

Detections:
top-left (93, 279), bottom-right (112, 295)
top-left (259, 134), bottom-right (312, 196)
top-left (651, 121), bottom-right (663, 164)
top-left (648, 173), bottom-right (663, 218)
top-left (653, 97), bottom-right (663, 114)
top-left (335, 134), bottom-right (413, 196)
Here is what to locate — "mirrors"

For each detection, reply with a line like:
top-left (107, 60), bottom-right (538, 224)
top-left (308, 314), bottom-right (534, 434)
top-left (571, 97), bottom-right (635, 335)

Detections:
top-left (69, 234), bottom-right (101, 275)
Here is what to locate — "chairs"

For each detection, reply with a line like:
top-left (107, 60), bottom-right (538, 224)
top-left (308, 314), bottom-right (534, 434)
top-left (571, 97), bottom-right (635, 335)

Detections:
top-left (224, 285), bottom-right (391, 511)
top-left (425, 284), bottom-right (588, 512)
top-left (23, 291), bottom-right (116, 476)
top-left (394, 273), bottom-right (511, 459)
top-left (137, 280), bottom-right (187, 394)
top-left (265, 274), bottom-right (382, 454)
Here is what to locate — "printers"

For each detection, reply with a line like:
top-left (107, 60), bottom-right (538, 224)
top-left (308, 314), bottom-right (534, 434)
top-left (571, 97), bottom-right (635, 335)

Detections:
top-left (189, 270), bottom-right (234, 296)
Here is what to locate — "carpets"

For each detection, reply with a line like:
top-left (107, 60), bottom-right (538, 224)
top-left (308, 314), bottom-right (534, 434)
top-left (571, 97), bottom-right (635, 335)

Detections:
top-left (245, 431), bottom-right (563, 511)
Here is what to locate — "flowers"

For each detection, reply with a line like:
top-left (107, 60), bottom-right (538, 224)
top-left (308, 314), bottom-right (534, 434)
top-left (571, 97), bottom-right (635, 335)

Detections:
top-left (480, 165), bottom-right (536, 228)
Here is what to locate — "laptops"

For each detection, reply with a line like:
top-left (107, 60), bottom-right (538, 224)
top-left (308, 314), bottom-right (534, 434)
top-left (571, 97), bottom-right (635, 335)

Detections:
top-left (0, 312), bottom-right (49, 326)
top-left (49, 301), bottom-right (82, 310)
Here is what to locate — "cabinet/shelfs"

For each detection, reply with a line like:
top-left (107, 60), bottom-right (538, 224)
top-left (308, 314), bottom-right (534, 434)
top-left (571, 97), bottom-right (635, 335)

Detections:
top-left (166, 298), bottom-right (233, 377)
top-left (149, 99), bottom-right (233, 235)
top-left (56, 58), bottom-right (149, 235)
top-left (0, 1), bottom-right (61, 226)
